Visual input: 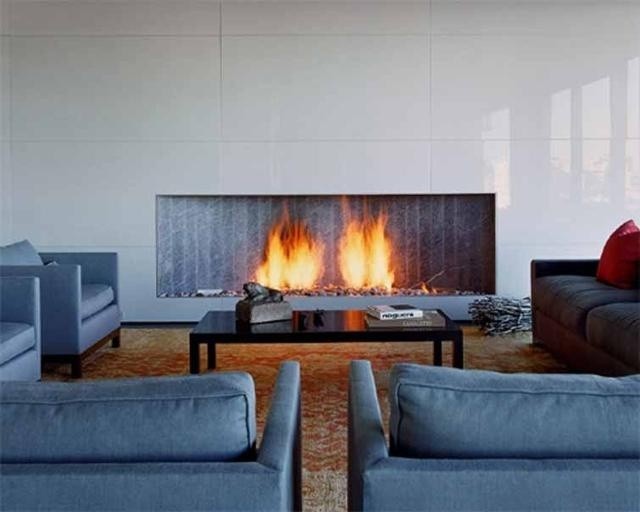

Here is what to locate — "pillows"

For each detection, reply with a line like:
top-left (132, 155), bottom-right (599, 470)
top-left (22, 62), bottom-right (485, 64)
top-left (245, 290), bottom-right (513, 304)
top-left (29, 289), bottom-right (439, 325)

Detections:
top-left (0, 239), bottom-right (44, 266)
top-left (596, 220), bottom-right (640, 289)
top-left (389, 363), bottom-right (640, 459)
top-left (0, 370), bottom-right (256, 463)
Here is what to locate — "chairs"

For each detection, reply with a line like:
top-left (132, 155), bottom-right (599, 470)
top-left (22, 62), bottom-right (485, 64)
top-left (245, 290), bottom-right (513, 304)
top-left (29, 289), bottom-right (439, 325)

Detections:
top-left (0, 275), bottom-right (43, 381)
top-left (0, 239), bottom-right (123, 378)
top-left (347, 359), bottom-right (640, 512)
top-left (0, 361), bottom-right (303, 512)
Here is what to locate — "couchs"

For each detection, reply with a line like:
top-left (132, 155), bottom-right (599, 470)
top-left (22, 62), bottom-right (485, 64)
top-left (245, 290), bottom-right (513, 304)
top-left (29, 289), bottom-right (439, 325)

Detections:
top-left (531, 221), bottom-right (640, 376)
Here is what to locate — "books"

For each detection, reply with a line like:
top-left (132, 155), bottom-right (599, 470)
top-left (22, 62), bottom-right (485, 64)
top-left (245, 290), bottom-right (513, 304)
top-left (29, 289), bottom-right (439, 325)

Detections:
top-left (362, 304), bottom-right (447, 329)
